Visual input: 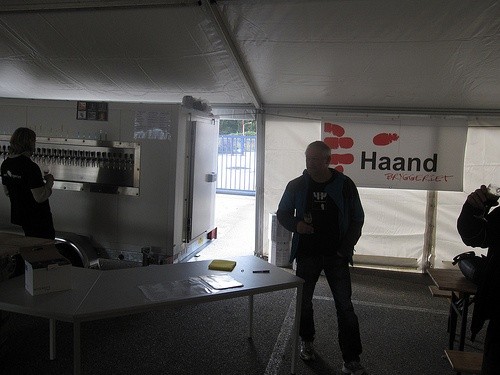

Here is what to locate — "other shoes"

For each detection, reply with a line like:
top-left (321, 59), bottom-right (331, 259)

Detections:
top-left (342, 362), bottom-right (367, 375)
top-left (299, 340), bottom-right (316, 361)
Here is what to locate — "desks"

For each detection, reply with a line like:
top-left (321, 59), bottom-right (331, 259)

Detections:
top-left (427, 267), bottom-right (478, 352)
top-left (1, 237), bottom-right (305, 375)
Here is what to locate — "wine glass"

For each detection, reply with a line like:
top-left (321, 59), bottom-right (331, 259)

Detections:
top-left (303, 213), bottom-right (314, 234)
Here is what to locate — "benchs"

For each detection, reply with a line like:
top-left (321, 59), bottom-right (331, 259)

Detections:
top-left (429, 285), bottom-right (474, 334)
top-left (444, 349), bottom-right (484, 375)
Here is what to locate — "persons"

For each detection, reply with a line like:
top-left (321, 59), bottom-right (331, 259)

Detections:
top-left (0, 127), bottom-right (56, 240)
top-left (457, 185), bottom-right (500, 375)
top-left (277, 141), bottom-right (368, 375)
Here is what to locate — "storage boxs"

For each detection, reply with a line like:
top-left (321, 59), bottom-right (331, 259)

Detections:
top-left (20, 245), bottom-right (73, 296)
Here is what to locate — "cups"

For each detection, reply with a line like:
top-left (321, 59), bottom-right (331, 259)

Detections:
top-left (43, 167), bottom-right (49, 176)
top-left (485, 184), bottom-right (500, 207)
